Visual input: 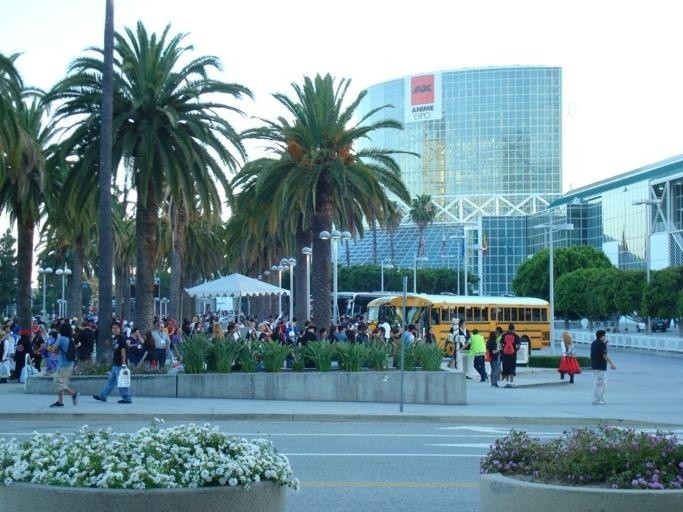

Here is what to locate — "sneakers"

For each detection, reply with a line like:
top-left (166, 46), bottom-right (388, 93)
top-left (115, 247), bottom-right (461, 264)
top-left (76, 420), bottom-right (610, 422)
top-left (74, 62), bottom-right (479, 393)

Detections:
top-left (72, 391), bottom-right (80, 406)
top-left (49, 401), bottom-right (65, 408)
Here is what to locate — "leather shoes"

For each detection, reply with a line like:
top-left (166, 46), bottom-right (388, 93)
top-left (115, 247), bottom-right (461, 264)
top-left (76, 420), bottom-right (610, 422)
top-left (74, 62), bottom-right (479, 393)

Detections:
top-left (118, 400), bottom-right (132, 404)
top-left (93, 394), bottom-right (106, 401)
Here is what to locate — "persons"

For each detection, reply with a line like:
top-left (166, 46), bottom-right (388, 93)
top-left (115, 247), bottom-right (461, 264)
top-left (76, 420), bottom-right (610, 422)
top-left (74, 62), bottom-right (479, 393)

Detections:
top-left (558, 331), bottom-right (575, 383)
top-left (92, 322), bottom-right (132, 403)
top-left (445, 318), bottom-right (519, 388)
top-left (46, 322), bottom-right (80, 407)
top-left (112, 312), bottom-right (438, 371)
top-left (1, 311), bottom-right (98, 383)
top-left (590, 329), bottom-right (617, 405)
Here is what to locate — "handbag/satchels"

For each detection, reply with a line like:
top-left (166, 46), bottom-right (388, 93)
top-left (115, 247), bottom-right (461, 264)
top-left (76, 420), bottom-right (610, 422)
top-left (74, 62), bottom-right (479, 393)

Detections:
top-left (0, 360), bottom-right (11, 378)
top-left (19, 353), bottom-right (33, 384)
top-left (117, 365), bottom-right (131, 388)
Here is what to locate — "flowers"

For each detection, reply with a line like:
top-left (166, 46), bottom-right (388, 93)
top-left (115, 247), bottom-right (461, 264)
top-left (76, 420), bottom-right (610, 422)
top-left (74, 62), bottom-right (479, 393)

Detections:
top-left (0, 417), bottom-right (301, 495)
top-left (479, 418), bottom-right (683, 490)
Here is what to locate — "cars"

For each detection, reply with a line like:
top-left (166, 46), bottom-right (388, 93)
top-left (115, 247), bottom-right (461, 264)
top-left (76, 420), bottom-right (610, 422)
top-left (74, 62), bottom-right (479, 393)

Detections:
top-left (618, 312), bottom-right (666, 334)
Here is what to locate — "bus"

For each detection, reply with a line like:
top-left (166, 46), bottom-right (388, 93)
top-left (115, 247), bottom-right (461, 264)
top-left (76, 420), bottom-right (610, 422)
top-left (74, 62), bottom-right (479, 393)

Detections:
top-left (364, 297), bottom-right (395, 340)
top-left (328, 292), bottom-right (355, 324)
top-left (384, 294), bottom-right (551, 358)
top-left (345, 291), bottom-right (456, 327)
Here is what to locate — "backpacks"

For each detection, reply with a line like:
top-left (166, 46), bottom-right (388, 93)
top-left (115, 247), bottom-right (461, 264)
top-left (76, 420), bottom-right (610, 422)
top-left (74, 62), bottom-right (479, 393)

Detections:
top-left (63, 336), bottom-right (76, 361)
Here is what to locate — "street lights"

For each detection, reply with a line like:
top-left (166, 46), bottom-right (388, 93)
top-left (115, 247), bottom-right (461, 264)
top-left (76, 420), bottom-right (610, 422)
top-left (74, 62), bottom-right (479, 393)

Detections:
top-left (448, 235), bottom-right (467, 296)
top-left (36, 266), bottom-right (54, 316)
top-left (380, 258), bottom-right (392, 292)
top-left (301, 247), bottom-right (316, 321)
top-left (631, 197), bottom-right (664, 333)
top-left (56, 262), bottom-right (73, 319)
top-left (254, 263), bottom-right (289, 319)
top-left (56, 298), bottom-right (69, 319)
top-left (548, 208), bottom-right (574, 356)
top-left (153, 296), bottom-right (170, 320)
top-left (411, 251), bottom-right (429, 293)
top-left (281, 256), bottom-right (297, 323)
top-left (319, 228), bottom-right (352, 327)
